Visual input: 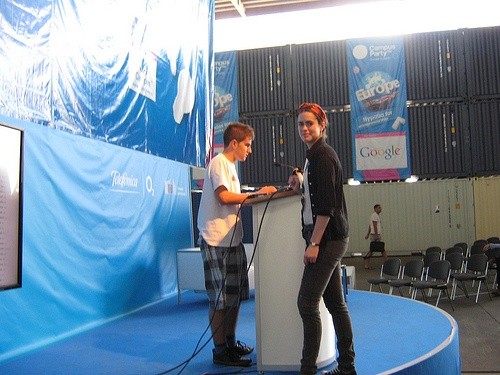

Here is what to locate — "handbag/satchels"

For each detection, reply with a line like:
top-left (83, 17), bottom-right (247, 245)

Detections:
top-left (370, 239), bottom-right (384, 252)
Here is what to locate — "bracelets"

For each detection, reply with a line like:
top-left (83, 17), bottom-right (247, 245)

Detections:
top-left (310, 242), bottom-right (320, 246)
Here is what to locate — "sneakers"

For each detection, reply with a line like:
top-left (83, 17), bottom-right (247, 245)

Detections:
top-left (214, 347), bottom-right (252, 365)
top-left (322, 368), bottom-right (356, 375)
top-left (233, 340), bottom-right (254, 355)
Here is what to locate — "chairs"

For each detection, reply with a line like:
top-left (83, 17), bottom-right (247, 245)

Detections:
top-left (368, 237), bottom-right (500, 312)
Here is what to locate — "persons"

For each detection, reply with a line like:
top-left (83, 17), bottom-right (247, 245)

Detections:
top-left (364, 204), bottom-right (389, 270)
top-left (196, 122), bottom-right (278, 367)
top-left (483, 243), bottom-right (500, 296)
top-left (288, 103), bottom-right (358, 375)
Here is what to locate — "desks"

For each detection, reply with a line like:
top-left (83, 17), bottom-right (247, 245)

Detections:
top-left (176, 246), bottom-right (205, 301)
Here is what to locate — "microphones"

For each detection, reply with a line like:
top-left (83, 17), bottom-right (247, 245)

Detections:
top-left (274, 162), bottom-right (295, 169)
